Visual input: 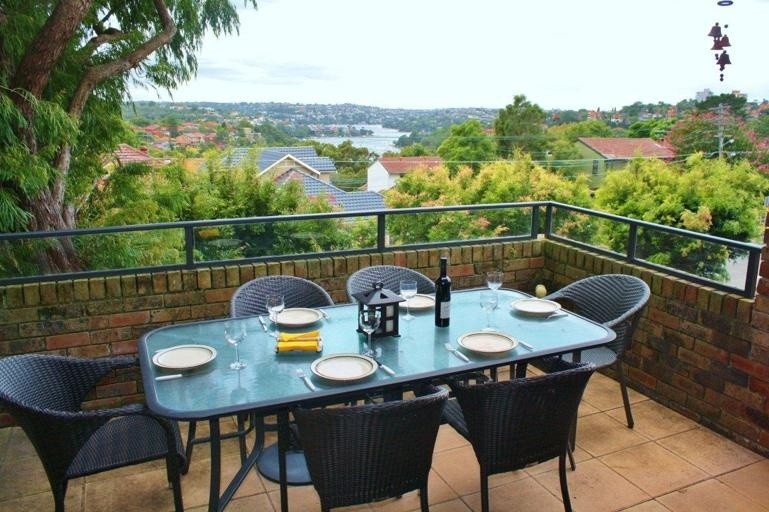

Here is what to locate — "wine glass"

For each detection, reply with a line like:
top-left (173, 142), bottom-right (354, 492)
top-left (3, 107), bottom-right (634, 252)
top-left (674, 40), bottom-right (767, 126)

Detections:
top-left (359, 310), bottom-right (381, 359)
top-left (265, 293), bottom-right (286, 337)
top-left (222, 317), bottom-right (248, 370)
top-left (479, 289), bottom-right (499, 331)
top-left (399, 279), bottom-right (418, 320)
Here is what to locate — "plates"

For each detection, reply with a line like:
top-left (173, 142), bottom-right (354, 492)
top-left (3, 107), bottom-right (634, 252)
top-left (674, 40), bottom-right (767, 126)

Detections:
top-left (309, 352), bottom-right (377, 383)
top-left (456, 330), bottom-right (520, 355)
top-left (270, 308), bottom-right (324, 328)
top-left (509, 298), bottom-right (562, 317)
top-left (152, 344), bottom-right (217, 369)
top-left (396, 294), bottom-right (436, 311)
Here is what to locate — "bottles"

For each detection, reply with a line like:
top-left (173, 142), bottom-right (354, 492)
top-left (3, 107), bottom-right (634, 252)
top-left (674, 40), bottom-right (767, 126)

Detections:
top-left (433, 257), bottom-right (453, 329)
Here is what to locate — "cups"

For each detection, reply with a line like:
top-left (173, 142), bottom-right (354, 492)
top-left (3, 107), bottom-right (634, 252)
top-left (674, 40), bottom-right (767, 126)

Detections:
top-left (486, 270), bottom-right (505, 290)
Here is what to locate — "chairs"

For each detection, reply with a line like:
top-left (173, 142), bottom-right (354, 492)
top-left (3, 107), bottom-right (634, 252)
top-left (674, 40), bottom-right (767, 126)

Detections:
top-left (346, 264), bottom-right (437, 405)
top-left (440, 361), bottom-right (599, 511)
top-left (539, 273), bottom-right (651, 451)
top-left (278, 384), bottom-right (449, 510)
top-left (231, 275), bottom-right (336, 465)
top-left (0, 354), bottom-right (188, 512)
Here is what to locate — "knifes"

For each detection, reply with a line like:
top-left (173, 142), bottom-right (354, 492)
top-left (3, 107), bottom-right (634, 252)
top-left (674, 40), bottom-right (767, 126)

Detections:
top-left (154, 368), bottom-right (214, 382)
top-left (372, 355), bottom-right (396, 376)
top-left (259, 315), bottom-right (268, 333)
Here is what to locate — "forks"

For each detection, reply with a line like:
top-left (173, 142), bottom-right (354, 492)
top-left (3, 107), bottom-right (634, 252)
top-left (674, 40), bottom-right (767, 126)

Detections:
top-left (295, 367), bottom-right (317, 392)
top-left (320, 309), bottom-right (336, 325)
top-left (536, 313), bottom-right (569, 321)
top-left (444, 342), bottom-right (469, 363)
